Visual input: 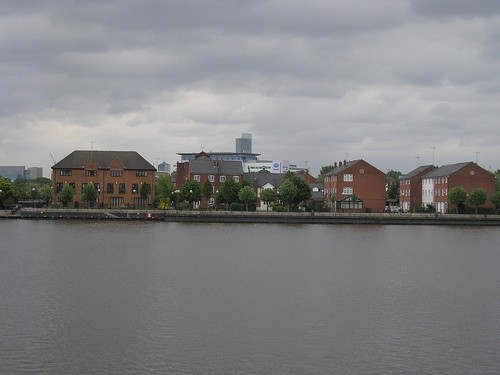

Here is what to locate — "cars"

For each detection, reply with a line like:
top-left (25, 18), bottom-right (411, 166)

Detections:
top-left (385, 205), bottom-right (401, 213)
